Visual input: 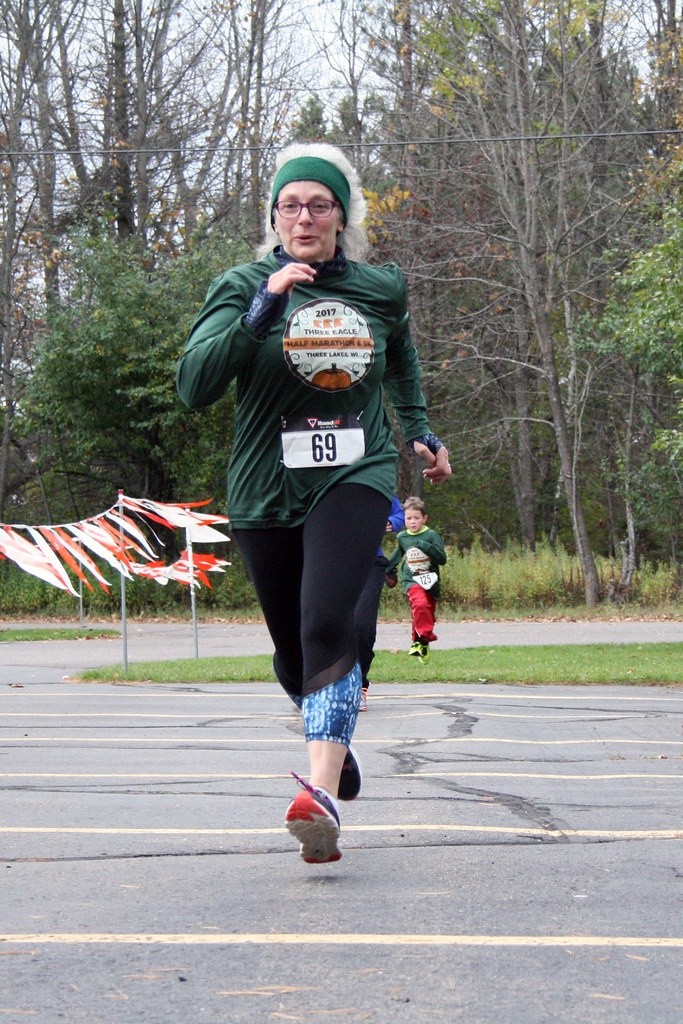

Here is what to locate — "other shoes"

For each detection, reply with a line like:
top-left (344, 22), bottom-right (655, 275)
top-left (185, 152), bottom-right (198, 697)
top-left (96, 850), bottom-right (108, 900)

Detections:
top-left (356, 688), bottom-right (368, 711)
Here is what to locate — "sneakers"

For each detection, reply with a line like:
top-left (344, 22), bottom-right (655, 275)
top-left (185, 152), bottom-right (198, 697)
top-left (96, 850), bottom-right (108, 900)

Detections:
top-left (418, 643), bottom-right (431, 666)
top-left (409, 642), bottom-right (421, 657)
top-left (338, 743), bottom-right (363, 801)
top-left (284, 771), bottom-right (342, 864)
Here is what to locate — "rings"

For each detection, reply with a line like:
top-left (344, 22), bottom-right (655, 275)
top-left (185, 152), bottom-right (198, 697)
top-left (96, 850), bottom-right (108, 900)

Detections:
top-left (448, 464), bottom-right (451, 466)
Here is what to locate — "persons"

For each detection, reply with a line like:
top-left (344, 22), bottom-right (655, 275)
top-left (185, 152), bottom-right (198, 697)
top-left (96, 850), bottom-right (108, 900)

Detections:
top-left (384, 494), bottom-right (447, 666)
top-left (353, 492), bottom-right (405, 713)
top-left (173, 141), bottom-right (453, 864)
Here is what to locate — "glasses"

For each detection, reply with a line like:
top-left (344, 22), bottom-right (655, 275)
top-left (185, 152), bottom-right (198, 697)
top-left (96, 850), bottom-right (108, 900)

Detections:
top-left (273, 199), bottom-right (340, 218)
